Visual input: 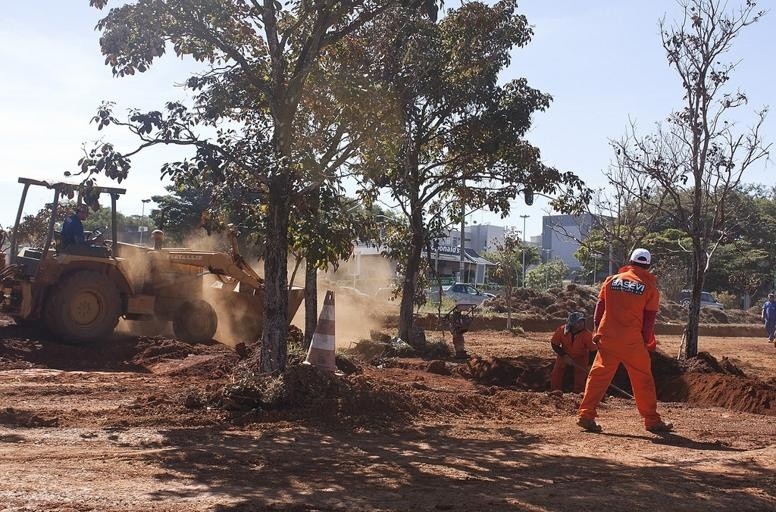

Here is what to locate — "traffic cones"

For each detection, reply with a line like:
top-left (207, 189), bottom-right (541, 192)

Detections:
top-left (298, 287), bottom-right (347, 382)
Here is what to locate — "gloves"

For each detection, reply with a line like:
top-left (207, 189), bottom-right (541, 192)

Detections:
top-left (560, 354), bottom-right (573, 366)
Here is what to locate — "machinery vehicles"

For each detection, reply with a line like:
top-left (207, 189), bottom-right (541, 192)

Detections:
top-left (0, 178), bottom-right (303, 360)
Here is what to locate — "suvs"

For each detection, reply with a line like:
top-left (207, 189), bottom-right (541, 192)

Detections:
top-left (679, 288), bottom-right (725, 314)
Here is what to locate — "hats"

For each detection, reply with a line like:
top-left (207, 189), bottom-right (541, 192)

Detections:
top-left (629, 248), bottom-right (651, 264)
top-left (77, 205), bottom-right (90, 215)
top-left (568, 312), bottom-right (586, 324)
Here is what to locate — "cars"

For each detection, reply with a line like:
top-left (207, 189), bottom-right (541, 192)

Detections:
top-left (373, 279), bottom-right (496, 308)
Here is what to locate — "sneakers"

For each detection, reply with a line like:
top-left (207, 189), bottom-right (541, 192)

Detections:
top-left (647, 419), bottom-right (673, 431)
top-left (576, 417), bottom-right (601, 432)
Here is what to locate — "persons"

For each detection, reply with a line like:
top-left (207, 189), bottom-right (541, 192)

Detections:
top-left (574, 247), bottom-right (672, 433)
top-left (58, 204), bottom-right (108, 258)
top-left (551, 311), bottom-right (597, 392)
top-left (762, 292), bottom-right (776, 343)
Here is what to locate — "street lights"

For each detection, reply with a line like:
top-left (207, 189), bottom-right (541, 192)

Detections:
top-left (520, 214), bottom-right (532, 288)
top-left (476, 221), bottom-right (493, 256)
top-left (139, 197), bottom-right (152, 246)
